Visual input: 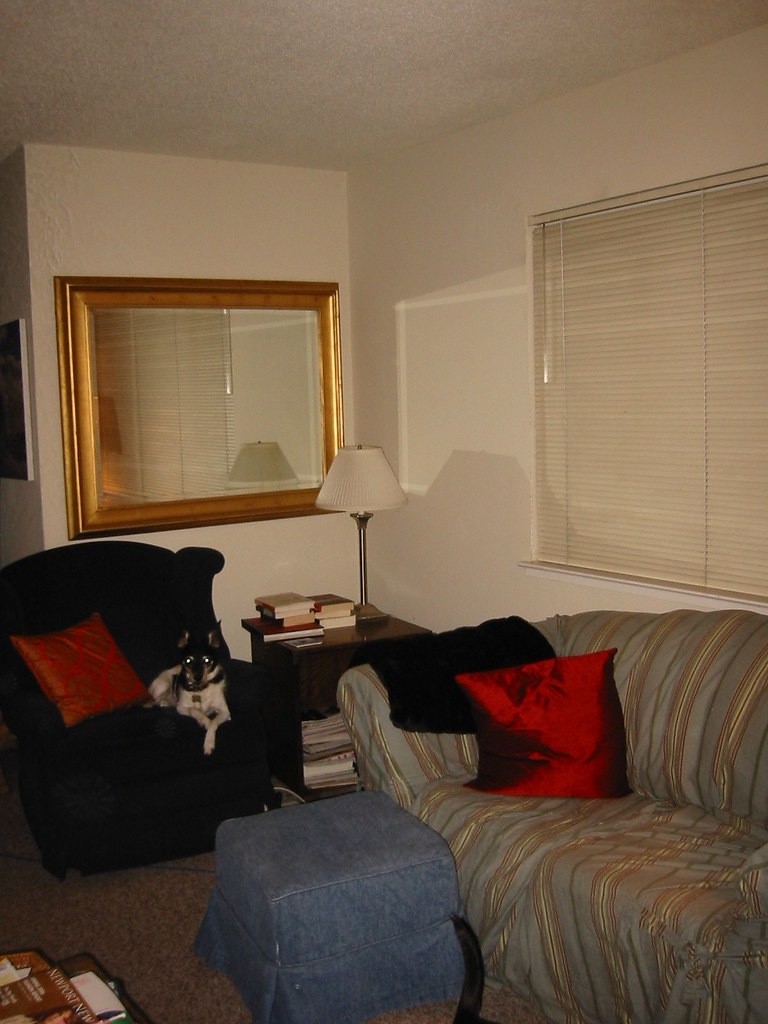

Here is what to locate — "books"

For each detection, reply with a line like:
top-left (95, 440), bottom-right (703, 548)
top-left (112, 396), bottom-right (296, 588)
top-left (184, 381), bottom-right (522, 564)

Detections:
top-left (242, 593), bottom-right (360, 790)
top-left (0, 952), bottom-right (133, 1024)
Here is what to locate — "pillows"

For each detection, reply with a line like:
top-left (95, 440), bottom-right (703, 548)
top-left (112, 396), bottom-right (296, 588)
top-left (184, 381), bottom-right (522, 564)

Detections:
top-left (10, 612), bottom-right (155, 729)
top-left (456, 647), bottom-right (634, 799)
top-left (348, 617), bottom-right (557, 733)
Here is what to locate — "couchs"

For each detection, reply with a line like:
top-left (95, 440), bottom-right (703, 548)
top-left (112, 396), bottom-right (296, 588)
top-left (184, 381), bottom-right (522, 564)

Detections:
top-left (0, 542), bottom-right (297, 880)
top-left (337, 611), bottom-right (768, 1024)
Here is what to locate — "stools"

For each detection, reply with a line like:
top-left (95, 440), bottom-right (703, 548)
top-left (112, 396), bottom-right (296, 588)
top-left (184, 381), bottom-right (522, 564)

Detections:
top-left (194, 790), bottom-right (472, 1024)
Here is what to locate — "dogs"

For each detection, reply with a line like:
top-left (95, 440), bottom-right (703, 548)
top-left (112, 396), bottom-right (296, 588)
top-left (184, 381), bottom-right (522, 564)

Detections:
top-left (143, 618), bottom-right (230, 756)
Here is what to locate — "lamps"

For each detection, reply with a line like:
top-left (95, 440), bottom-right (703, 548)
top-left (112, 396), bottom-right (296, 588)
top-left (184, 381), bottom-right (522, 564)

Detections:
top-left (315, 445), bottom-right (409, 621)
top-left (229, 441), bottom-right (300, 492)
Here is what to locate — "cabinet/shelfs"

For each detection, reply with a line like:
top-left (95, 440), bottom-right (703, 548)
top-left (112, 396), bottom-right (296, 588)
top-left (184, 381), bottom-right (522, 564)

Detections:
top-left (251, 617), bottom-right (432, 797)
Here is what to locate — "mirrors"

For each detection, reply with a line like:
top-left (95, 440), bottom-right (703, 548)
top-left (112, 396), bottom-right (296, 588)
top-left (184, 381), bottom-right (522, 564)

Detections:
top-left (53, 275), bottom-right (347, 541)
